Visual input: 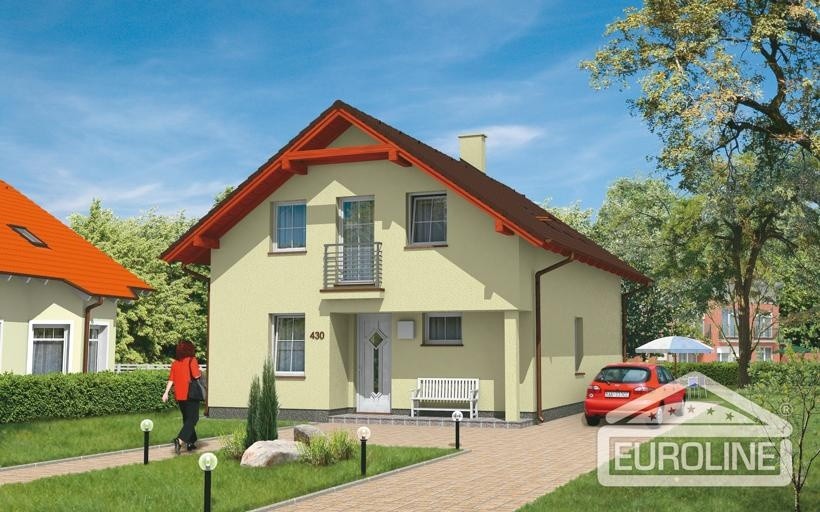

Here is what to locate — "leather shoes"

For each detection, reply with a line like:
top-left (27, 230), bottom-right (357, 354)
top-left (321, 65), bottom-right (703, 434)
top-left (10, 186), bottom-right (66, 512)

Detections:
top-left (175, 438), bottom-right (182, 456)
top-left (187, 443), bottom-right (197, 451)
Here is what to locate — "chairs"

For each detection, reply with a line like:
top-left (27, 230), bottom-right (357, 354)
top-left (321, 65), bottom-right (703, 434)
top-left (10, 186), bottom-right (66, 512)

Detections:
top-left (688, 377), bottom-right (707, 400)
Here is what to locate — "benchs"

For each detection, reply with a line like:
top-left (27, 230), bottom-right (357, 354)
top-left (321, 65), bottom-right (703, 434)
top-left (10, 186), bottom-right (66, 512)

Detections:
top-left (411, 378), bottom-right (479, 419)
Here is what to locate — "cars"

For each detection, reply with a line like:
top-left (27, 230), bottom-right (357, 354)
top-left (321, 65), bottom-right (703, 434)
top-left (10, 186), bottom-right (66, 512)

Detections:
top-left (584, 362), bottom-right (686, 430)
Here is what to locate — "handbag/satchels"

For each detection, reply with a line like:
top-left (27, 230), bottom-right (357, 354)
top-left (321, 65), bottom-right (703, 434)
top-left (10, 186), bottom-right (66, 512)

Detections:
top-left (187, 357), bottom-right (206, 401)
